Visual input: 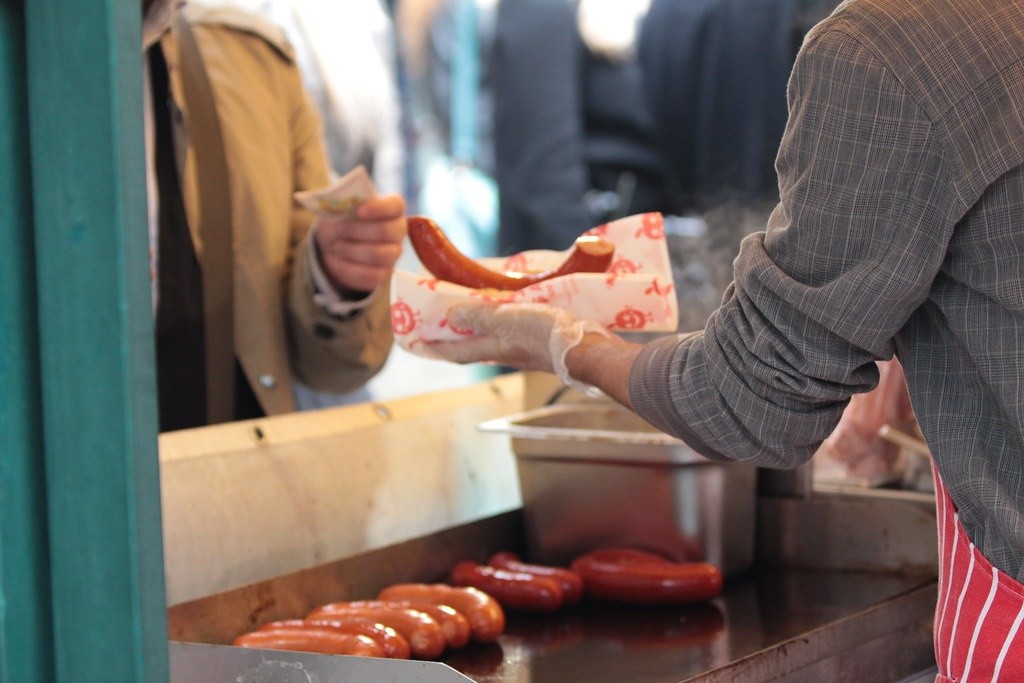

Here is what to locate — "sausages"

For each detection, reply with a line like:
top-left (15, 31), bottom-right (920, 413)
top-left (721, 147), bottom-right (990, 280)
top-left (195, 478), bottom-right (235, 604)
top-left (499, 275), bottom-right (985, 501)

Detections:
top-left (406, 216), bottom-right (614, 291)
top-left (232, 545), bottom-right (720, 660)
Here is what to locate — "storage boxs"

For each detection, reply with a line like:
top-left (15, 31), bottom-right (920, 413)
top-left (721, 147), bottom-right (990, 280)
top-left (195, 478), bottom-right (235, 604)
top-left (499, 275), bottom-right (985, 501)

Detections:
top-left (475, 405), bottom-right (762, 590)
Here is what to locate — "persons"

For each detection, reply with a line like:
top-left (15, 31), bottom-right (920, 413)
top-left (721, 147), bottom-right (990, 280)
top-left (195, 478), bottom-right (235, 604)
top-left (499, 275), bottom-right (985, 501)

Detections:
top-left (141, 0), bottom-right (1024, 683)
